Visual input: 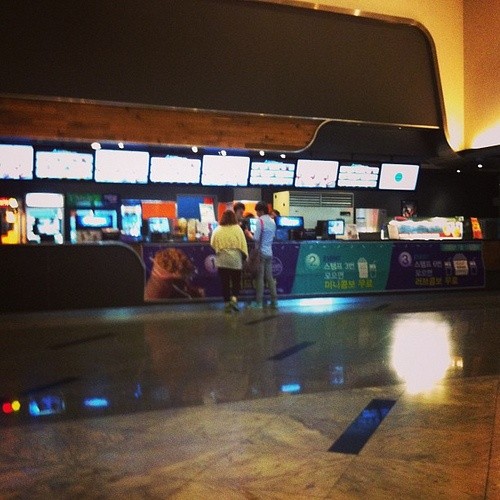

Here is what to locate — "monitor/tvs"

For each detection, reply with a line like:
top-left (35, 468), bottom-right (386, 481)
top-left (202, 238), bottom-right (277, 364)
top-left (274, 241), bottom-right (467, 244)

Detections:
top-left (148, 218), bottom-right (170, 233)
top-left (328, 220), bottom-right (344, 235)
top-left (0, 141), bottom-right (421, 191)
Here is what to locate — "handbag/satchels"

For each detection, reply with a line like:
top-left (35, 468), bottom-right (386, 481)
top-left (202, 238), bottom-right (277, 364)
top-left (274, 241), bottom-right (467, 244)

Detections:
top-left (245, 248), bottom-right (260, 275)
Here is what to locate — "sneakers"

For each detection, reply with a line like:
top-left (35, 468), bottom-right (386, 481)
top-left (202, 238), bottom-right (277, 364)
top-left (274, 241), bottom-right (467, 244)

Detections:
top-left (229, 300), bottom-right (239, 311)
top-left (224, 304), bottom-right (231, 312)
top-left (267, 304), bottom-right (278, 308)
top-left (248, 301), bottom-right (263, 309)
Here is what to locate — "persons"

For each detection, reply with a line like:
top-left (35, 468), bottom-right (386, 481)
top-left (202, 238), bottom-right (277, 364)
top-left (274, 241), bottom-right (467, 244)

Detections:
top-left (233, 202), bottom-right (251, 241)
top-left (244, 199), bottom-right (280, 310)
top-left (269, 210), bottom-right (280, 221)
top-left (209, 211), bottom-right (249, 314)
